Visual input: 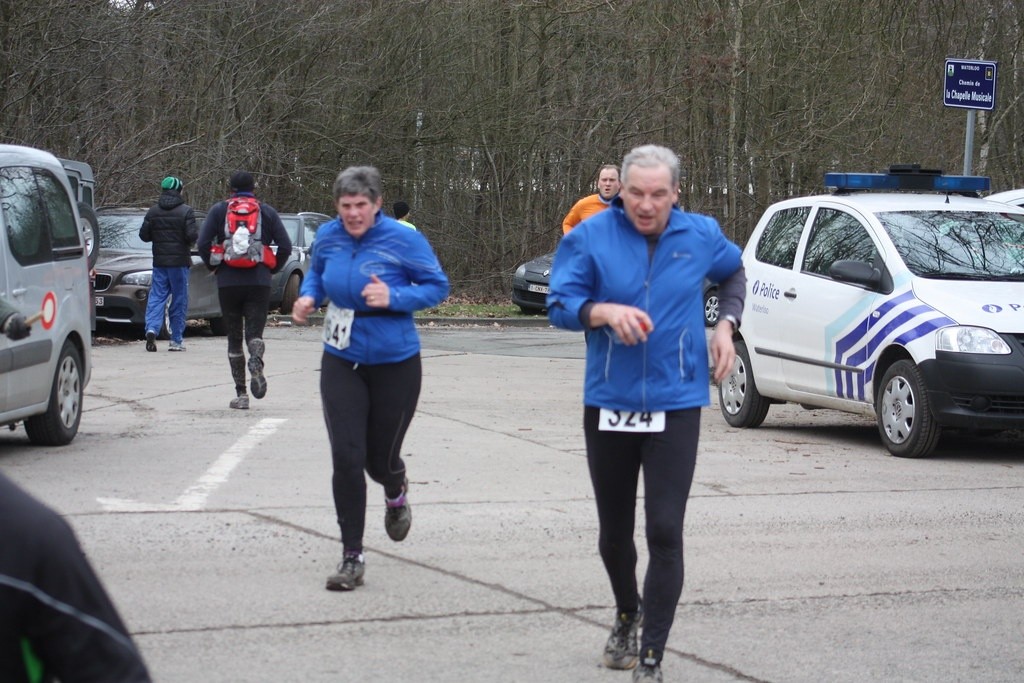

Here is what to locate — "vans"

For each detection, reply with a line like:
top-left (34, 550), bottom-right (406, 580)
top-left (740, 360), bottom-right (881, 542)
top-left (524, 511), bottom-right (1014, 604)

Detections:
top-left (0, 143), bottom-right (93, 448)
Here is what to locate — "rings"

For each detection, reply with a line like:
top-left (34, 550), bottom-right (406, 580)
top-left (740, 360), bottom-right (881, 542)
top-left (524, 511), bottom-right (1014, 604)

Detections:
top-left (370, 296), bottom-right (375, 301)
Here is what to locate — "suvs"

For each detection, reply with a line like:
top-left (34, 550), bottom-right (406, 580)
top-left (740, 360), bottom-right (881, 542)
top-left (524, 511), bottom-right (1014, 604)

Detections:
top-left (31, 158), bottom-right (101, 273)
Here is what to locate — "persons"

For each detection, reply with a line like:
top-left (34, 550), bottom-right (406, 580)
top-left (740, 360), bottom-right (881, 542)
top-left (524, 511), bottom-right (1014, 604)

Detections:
top-left (139, 177), bottom-right (198, 352)
top-left (0, 472), bottom-right (152, 683)
top-left (292, 166), bottom-right (449, 590)
top-left (198, 172), bottom-right (292, 409)
top-left (394, 202), bottom-right (416, 230)
top-left (563, 165), bottom-right (622, 235)
top-left (546, 145), bottom-right (747, 683)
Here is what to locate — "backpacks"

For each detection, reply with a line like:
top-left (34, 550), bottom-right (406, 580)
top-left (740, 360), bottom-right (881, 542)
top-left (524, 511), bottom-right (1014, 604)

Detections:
top-left (209, 196), bottom-right (277, 268)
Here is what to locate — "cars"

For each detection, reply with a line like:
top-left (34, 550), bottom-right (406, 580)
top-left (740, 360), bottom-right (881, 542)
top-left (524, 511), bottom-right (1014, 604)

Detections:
top-left (511, 252), bottom-right (721, 329)
top-left (96, 202), bottom-right (229, 342)
top-left (269, 212), bottom-right (333, 316)
top-left (717, 162), bottom-right (1024, 460)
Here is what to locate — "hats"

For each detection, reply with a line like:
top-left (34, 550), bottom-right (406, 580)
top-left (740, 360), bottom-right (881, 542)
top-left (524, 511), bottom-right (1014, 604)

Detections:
top-left (394, 202), bottom-right (409, 218)
top-left (231, 170), bottom-right (255, 192)
top-left (162, 176), bottom-right (183, 193)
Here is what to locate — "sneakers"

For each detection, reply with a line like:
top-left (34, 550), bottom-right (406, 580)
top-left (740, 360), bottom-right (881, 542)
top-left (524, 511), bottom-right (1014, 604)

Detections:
top-left (146, 332), bottom-right (157, 352)
top-left (603, 594), bottom-right (644, 670)
top-left (385, 476), bottom-right (412, 542)
top-left (230, 394), bottom-right (249, 409)
top-left (248, 356), bottom-right (268, 398)
top-left (327, 551), bottom-right (364, 590)
top-left (168, 340), bottom-right (187, 351)
top-left (632, 664), bottom-right (662, 683)
